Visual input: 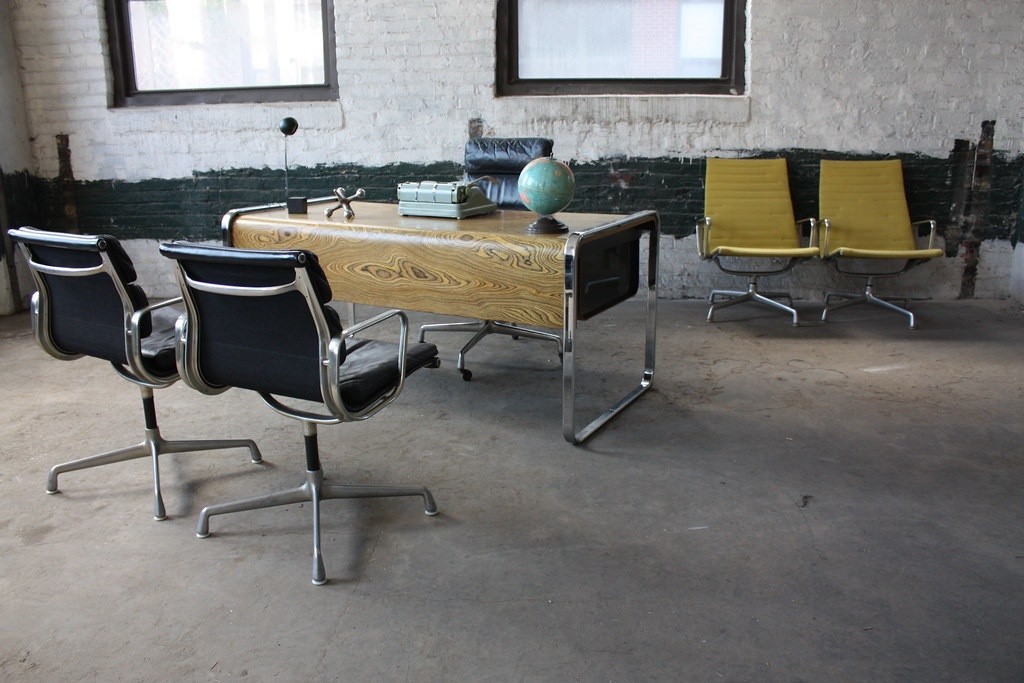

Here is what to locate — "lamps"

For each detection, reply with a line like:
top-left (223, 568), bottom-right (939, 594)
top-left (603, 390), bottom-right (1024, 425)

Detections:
top-left (278, 115), bottom-right (300, 203)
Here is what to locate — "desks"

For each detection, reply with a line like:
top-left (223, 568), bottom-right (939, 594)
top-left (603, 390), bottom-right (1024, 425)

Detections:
top-left (218, 195), bottom-right (664, 448)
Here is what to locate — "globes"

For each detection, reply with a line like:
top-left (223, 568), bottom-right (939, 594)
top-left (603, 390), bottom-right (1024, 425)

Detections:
top-left (516, 152), bottom-right (576, 235)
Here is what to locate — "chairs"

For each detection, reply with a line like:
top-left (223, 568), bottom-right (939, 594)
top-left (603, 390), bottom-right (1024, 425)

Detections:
top-left (8, 225), bottom-right (266, 524)
top-left (695, 155), bottom-right (818, 327)
top-left (816, 158), bottom-right (944, 331)
top-left (157, 239), bottom-right (441, 588)
top-left (417, 137), bottom-right (565, 383)
